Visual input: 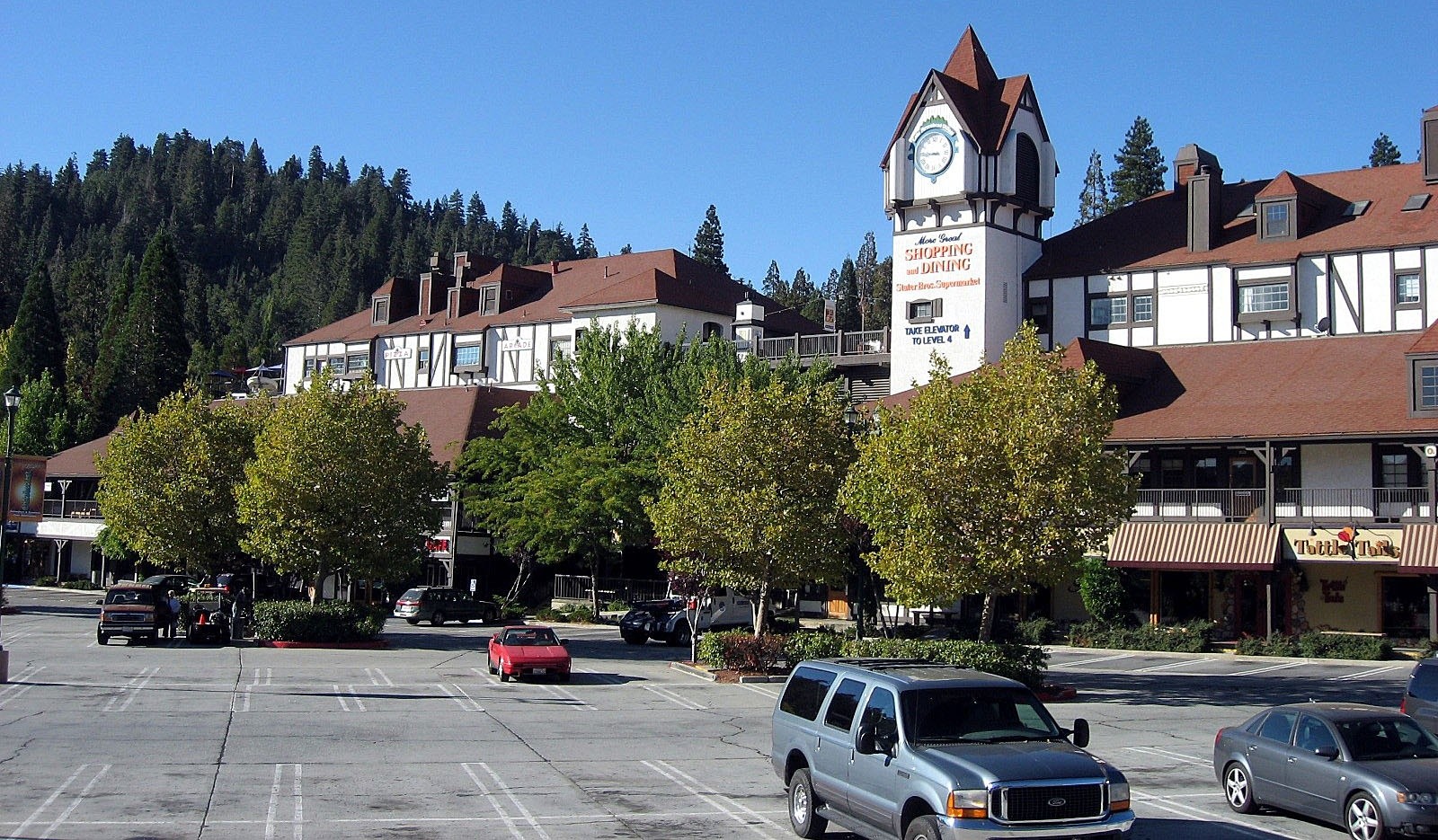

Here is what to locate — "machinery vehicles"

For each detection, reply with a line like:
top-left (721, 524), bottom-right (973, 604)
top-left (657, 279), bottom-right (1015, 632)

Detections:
top-left (183, 582), bottom-right (232, 645)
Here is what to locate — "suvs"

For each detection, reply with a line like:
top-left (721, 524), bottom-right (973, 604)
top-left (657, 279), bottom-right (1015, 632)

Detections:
top-left (215, 573), bottom-right (298, 604)
top-left (95, 581), bottom-right (161, 646)
top-left (619, 597), bottom-right (712, 648)
top-left (393, 584), bottom-right (500, 626)
top-left (770, 651), bottom-right (1138, 840)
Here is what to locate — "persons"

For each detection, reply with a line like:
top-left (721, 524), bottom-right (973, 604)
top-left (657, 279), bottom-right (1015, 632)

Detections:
top-left (162, 589), bottom-right (180, 642)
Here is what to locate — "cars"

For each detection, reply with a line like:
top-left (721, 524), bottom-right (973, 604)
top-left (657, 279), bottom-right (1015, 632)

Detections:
top-left (141, 575), bottom-right (201, 597)
top-left (1213, 702), bottom-right (1438, 840)
top-left (488, 625), bottom-right (572, 682)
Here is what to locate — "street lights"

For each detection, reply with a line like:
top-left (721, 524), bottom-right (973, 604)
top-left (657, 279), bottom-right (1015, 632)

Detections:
top-left (1, 384), bottom-right (25, 598)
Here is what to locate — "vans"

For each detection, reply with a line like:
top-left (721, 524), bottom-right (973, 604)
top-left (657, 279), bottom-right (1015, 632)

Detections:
top-left (1400, 657), bottom-right (1438, 740)
top-left (665, 571), bottom-right (775, 628)
